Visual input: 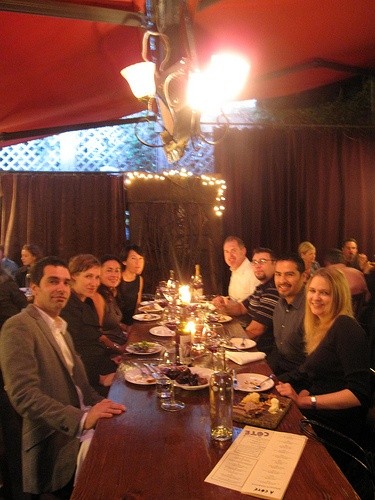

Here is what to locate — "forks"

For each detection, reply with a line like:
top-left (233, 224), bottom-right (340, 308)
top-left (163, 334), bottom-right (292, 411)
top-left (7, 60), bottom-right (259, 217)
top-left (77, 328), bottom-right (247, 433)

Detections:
top-left (241, 336), bottom-right (245, 347)
top-left (232, 368), bottom-right (237, 388)
top-left (133, 363), bottom-right (148, 378)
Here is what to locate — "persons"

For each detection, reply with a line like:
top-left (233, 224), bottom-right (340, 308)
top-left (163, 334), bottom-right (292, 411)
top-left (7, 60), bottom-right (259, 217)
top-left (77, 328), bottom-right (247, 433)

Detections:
top-left (0, 256), bottom-right (127, 500)
top-left (296, 236), bottom-right (375, 373)
top-left (93, 255), bottom-right (132, 347)
top-left (274, 265), bottom-right (375, 500)
top-left (257, 252), bottom-right (315, 379)
top-left (221, 234), bottom-right (263, 304)
top-left (0, 242), bottom-right (46, 329)
top-left (111, 244), bottom-right (145, 326)
top-left (59, 251), bottom-right (127, 399)
top-left (214, 246), bottom-right (280, 352)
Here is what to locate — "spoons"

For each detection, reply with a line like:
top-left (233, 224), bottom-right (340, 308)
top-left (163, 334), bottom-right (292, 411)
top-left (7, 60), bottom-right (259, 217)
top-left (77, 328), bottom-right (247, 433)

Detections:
top-left (253, 374), bottom-right (276, 389)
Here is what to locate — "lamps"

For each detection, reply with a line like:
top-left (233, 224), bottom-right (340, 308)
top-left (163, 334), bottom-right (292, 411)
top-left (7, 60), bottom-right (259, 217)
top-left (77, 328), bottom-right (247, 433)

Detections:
top-left (121, 21), bottom-right (252, 164)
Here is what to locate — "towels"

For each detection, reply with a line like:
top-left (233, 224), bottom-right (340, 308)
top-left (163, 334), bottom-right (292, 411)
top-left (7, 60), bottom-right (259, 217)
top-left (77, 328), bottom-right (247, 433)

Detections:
top-left (226, 351), bottom-right (267, 365)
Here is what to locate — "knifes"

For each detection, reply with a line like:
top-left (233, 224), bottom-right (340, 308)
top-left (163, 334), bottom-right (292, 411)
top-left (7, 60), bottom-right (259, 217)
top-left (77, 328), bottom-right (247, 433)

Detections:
top-left (143, 363), bottom-right (158, 378)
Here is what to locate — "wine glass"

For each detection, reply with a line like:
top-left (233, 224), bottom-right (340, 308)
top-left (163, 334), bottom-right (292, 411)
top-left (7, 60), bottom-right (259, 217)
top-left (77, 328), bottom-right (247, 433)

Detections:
top-left (162, 307), bottom-right (180, 354)
top-left (159, 344), bottom-right (187, 411)
top-left (203, 323), bottom-right (226, 370)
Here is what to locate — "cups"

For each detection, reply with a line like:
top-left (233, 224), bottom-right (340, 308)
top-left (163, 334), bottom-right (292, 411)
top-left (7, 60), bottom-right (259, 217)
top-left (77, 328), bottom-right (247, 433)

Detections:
top-left (155, 359), bottom-right (171, 398)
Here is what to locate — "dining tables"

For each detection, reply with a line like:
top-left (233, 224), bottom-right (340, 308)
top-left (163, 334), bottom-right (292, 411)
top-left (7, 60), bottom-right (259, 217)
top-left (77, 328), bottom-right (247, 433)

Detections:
top-left (69, 317), bottom-right (356, 500)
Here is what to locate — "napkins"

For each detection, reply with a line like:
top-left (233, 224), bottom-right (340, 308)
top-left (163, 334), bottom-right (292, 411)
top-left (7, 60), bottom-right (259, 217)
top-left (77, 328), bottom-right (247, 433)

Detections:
top-left (226, 350), bottom-right (266, 365)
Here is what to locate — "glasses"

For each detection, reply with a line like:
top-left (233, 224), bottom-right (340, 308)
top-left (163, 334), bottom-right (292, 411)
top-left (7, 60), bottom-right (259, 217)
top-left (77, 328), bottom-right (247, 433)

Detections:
top-left (250, 259), bottom-right (273, 266)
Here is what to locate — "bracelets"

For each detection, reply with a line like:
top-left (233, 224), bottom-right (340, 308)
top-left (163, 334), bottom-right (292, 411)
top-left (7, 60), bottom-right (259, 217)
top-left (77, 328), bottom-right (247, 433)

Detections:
top-left (310, 394), bottom-right (317, 410)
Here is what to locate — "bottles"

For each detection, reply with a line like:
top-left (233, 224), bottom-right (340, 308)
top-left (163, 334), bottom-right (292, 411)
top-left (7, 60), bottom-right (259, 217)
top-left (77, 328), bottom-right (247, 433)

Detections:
top-left (25, 267), bottom-right (31, 288)
top-left (193, 265), bottom-right (203, 296)
top-left (167, 270), bottom-right (176, 288)
top-left (209, 347), bottom-right (233, 441)
top-left (175, 314), bottom-right (192, 367)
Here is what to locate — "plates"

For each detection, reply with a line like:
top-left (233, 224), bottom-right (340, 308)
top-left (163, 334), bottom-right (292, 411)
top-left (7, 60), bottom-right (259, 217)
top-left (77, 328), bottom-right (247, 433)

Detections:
top-left (137, 306), bottom-right (164, 312)
top-left (173, 367), bottom-right (215, 390)
top-left (210, 314), bottom-right (232, 323)
top-left (220, 338), bottom-right (257, 349)
top-left (195, 295), bottom-right (216, 303)
top-left (124, 367), bottom-right (156, 385)
top-left (132, 314), bottom-right (161, 321)
top-left (190, 303), bottom-right (216, 310)
top-left (149, 326), bottom-right (175, 336)
top-left (139, 301), bottom-right (155, 305)
top-left (233, 373), bottom-right (274, 392)
top-left (125, 342), bottom-right (162, 354)
